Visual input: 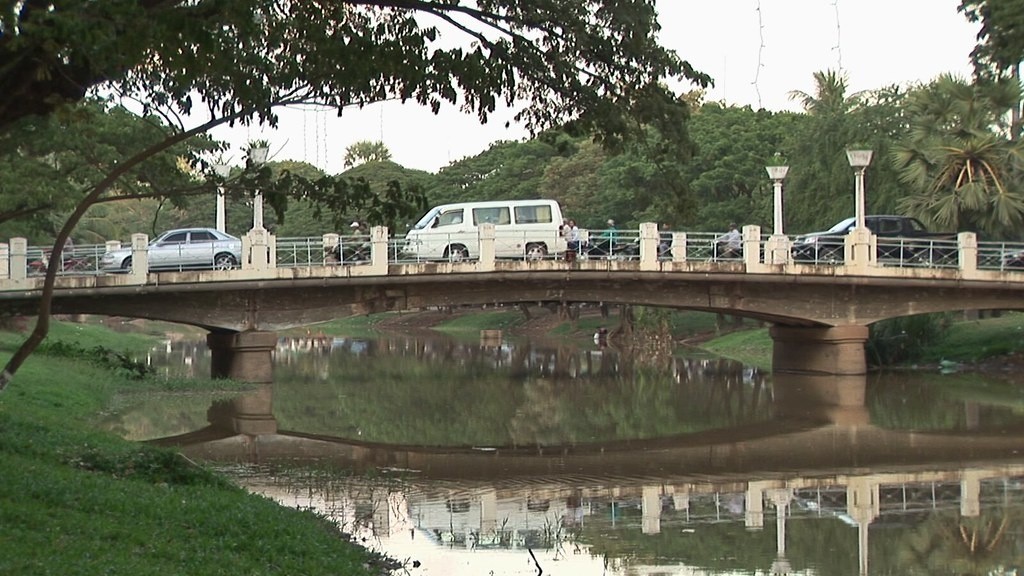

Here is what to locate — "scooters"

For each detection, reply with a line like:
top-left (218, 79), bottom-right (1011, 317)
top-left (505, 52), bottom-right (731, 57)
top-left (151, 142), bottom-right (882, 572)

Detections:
top-left (710, 237), bottom-right (742, 258)
top-left (583, 237), bottom-right (630, 261)
top-left (36, 250), bottom-right (77, 272)
top-left (324, 240), bottom-right (370, 265)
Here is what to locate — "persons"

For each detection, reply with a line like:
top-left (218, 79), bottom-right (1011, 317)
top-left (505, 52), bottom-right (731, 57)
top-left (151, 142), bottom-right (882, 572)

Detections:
top-left (563, 218), bottom-right (580, 261)
top-left (345, 222), bottom-right (371, 261)
top-left (60, 236), bottom-right (74, 271)
top-left (715, 223), bottom-right (741, 258)
top-left (443, 212), bottom-right (461, 225)
top-left (597, 219), bottom-right (616, 260)
top-left (657, 223), bottom-right (674, 257)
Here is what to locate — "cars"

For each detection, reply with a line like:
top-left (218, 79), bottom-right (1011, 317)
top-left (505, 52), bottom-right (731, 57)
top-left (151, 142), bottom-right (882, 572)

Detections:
top-left (101, 228), bottom-right (242, 271)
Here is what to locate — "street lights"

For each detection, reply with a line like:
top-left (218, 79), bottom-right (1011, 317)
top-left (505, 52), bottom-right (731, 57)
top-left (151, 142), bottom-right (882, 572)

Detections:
top-left (845, 141), bottom-right (874, 235)
top-left (765, 151), bottom-right (790, 242)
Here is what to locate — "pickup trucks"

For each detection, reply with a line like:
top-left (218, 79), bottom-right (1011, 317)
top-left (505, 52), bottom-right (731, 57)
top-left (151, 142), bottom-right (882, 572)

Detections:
top-left (790, 214), bottom-right (958, 265)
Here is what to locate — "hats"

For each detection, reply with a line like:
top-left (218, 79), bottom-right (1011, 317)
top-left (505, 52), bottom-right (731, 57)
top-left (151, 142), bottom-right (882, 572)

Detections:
top-left (350, 221), bottom-right (360, 228)
top-left (606, 219), bottom-right (615, 226)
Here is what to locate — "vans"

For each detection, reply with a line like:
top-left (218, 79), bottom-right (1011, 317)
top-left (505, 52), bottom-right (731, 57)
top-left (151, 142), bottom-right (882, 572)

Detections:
top-left (401, 199), bottom-right (567, 263)
